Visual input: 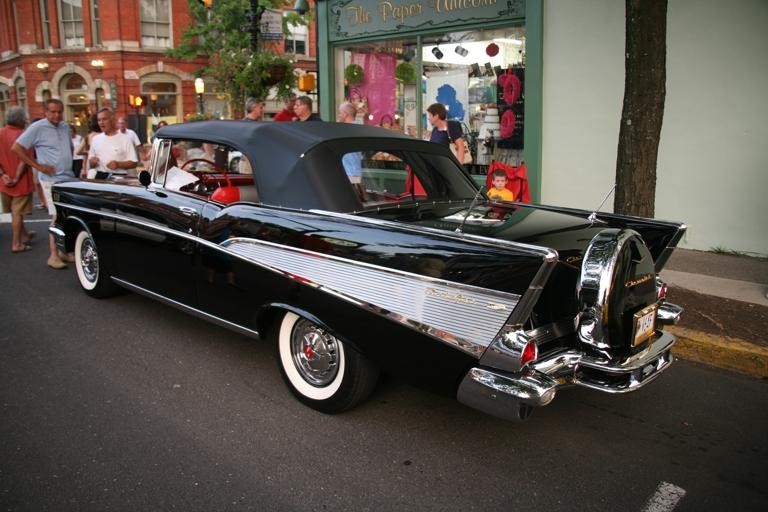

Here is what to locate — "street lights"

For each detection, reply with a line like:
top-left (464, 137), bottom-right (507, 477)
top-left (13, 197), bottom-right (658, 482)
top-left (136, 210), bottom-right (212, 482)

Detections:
top-left (193, 76), bottom-right (207, 116)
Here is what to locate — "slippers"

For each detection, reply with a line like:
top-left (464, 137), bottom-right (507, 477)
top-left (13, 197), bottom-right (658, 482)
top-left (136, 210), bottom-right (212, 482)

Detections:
top-left (23, 230), bottom-right (38, 242)
top-left (10, 243), bottom-right (33, 254)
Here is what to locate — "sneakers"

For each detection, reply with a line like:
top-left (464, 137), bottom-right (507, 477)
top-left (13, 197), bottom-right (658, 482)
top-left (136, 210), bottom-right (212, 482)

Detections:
top-left (47, 255), bottom-right (68, 269)
top-left (57, 251), bottom-right (75, 264)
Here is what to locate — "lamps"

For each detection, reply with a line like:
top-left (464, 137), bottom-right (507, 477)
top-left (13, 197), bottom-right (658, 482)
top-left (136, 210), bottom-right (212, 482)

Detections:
top-left (90, 60), bottom-right (104, 72)
top-left (431, 42), bottom-right (469, 60)
top-left (37, 61), bottom-right (49, 74)
top-left (298, 74), bottom-right (317, 96)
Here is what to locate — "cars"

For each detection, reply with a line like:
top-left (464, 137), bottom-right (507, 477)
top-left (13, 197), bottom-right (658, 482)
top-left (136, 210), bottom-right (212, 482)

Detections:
top-left (47, 118), bottom-right (691, 423)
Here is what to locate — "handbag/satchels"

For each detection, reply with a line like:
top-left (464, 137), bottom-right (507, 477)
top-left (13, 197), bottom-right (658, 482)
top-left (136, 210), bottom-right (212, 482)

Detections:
top-left (446, 119), bottom-right (474, 165)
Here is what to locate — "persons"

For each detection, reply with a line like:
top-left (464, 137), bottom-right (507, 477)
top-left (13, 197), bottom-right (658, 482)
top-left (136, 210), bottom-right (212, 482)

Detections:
top-left (426, 103), bottom-right (464, 166)
top-left (485, 168), bottom-right (513, 202)
top-left (242, 96), bottom-right (321, 122)
top-left (198, 143), bottom-right (216, 171)
top-left (336, 103), bottom-right (361, 184)
top-left (0, 99), bottom-right (168, 268)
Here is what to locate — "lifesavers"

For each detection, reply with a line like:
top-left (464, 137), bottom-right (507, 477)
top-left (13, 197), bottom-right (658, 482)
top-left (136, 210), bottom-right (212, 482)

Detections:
top-left (346, 63), bottom-right (364, 83)
top-left (502, 76), bottom-right (521, 105)
top-left (501, 109), bottom-right (516, 139)
